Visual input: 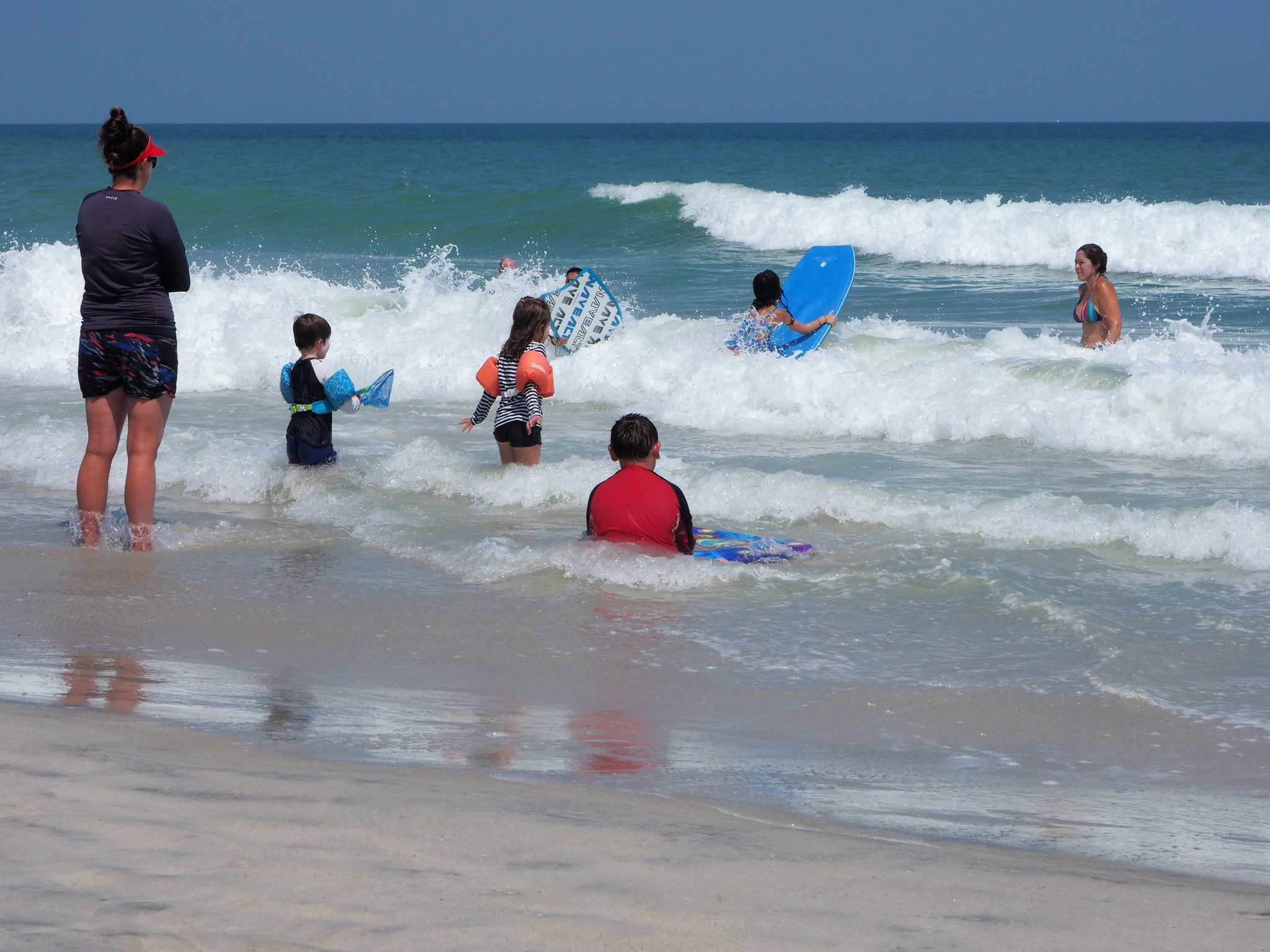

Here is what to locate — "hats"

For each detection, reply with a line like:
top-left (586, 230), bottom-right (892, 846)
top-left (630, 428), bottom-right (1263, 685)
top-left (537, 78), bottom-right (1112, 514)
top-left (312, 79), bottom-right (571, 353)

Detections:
top-left (108, 134), bottom-right (168, 169)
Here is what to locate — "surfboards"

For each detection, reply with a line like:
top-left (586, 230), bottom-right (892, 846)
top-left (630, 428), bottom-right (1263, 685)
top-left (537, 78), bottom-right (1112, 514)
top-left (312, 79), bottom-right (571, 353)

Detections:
top-left (768, 245), bottom-right (856, 360)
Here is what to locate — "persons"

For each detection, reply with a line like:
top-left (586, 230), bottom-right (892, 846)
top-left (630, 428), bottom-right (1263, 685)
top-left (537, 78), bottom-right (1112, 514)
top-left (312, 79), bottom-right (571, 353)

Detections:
top-left (1073, 243), bottom-right (1122, 349)
top-left (717, 269), bottom-right (837, 356)
top-left (74, 107), bottom-right (192, 548)
top-left (495, 258), bottom-right (521, 280)
top-left (547, 267), bottom-right (584, 346)
top-left (282, 313), bottom-right (363, 469)
top-left (585, 412), bottom-right (697, 556)
top-left (455, 295), bottom-right (554, 466)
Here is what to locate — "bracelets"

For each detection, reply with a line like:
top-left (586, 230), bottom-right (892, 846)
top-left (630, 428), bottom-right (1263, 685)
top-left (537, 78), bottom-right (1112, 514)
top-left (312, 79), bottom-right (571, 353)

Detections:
top-left (818, 318), bottom-right (823, 326)
top-left (824, 315), bottom-right (827, 324)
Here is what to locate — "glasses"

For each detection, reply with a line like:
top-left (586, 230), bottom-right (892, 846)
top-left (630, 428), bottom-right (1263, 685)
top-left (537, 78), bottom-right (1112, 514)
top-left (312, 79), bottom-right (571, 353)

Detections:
top-left (147, 156), bottom-right (157, 168)
